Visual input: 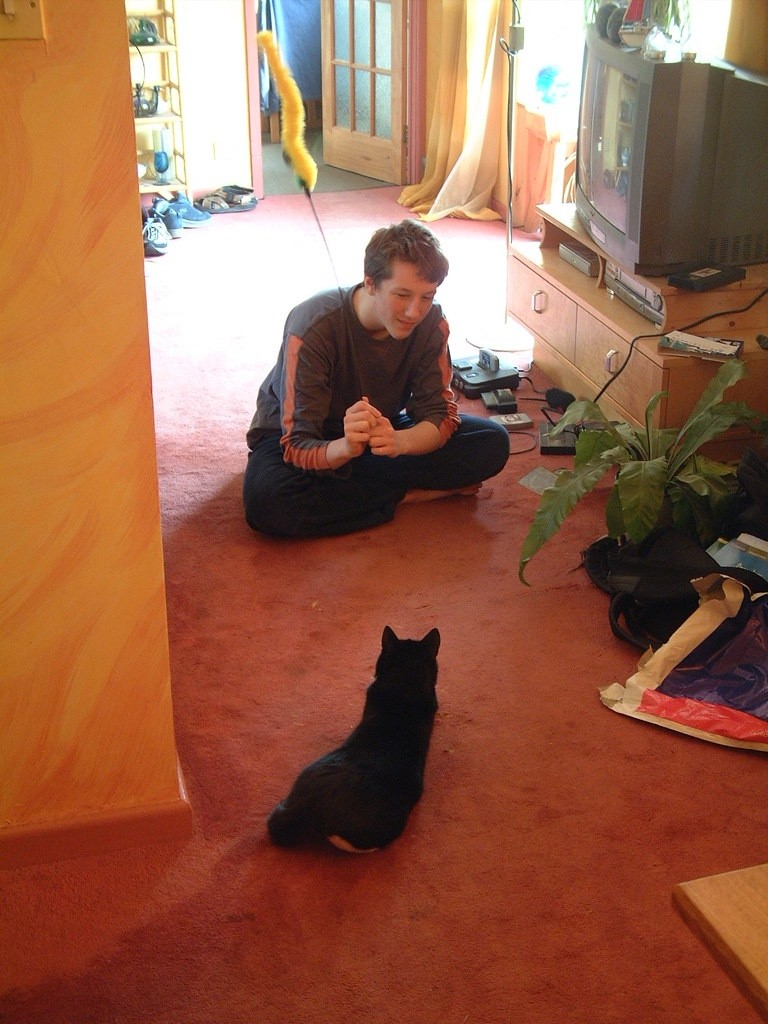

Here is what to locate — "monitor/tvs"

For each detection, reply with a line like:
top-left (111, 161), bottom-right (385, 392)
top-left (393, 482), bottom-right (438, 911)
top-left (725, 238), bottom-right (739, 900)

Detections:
top-left (571, 22), bottom-right (768, 275)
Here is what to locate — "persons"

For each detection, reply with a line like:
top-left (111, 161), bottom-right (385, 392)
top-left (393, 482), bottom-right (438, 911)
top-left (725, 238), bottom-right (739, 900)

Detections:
top-left (242, 218), bottom-right (510, 542)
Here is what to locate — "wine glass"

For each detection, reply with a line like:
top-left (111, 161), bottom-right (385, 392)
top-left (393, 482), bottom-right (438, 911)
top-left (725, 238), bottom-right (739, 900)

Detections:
top-left (154, 152), bottom-right (170, 186)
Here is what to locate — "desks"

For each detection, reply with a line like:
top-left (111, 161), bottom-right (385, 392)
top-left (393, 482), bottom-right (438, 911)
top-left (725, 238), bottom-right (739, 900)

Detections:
top-left (672, 864), bottom-right (767, 1024)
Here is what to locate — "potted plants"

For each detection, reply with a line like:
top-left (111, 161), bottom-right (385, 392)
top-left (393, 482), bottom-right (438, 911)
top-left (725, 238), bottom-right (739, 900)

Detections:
top-left (517, 358), bottom-right (768, 588)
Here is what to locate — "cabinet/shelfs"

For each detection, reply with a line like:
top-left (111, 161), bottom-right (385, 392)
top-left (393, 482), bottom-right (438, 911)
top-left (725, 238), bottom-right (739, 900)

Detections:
top-left (506, 203), bottom-right (768, 465)
top-left (126, 0), bottom-right (190, 202)
top-left (613, 75), bottom-right (637, 186)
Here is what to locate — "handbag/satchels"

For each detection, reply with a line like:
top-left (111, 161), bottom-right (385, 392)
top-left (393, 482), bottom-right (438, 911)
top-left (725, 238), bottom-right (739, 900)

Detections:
top-left (583, 527), bottom-right (720, 651)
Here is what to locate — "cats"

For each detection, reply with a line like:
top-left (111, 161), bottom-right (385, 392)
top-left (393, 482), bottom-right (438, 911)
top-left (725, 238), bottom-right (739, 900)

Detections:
top-left (265, 624), bottom-right (445, 852)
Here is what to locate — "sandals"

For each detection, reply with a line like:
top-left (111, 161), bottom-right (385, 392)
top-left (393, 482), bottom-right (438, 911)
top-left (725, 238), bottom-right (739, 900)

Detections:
top-left (194, 185), bottom-right (257, 213)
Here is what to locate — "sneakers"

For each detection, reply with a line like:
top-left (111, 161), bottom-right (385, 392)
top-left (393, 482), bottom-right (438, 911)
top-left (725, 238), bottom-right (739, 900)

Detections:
top-left (142, 218), bottom-right (172, 254)
top-left (170, 194), bottom-right (212, 228)
top-left (151, 200), bottom-right (183, 238)
